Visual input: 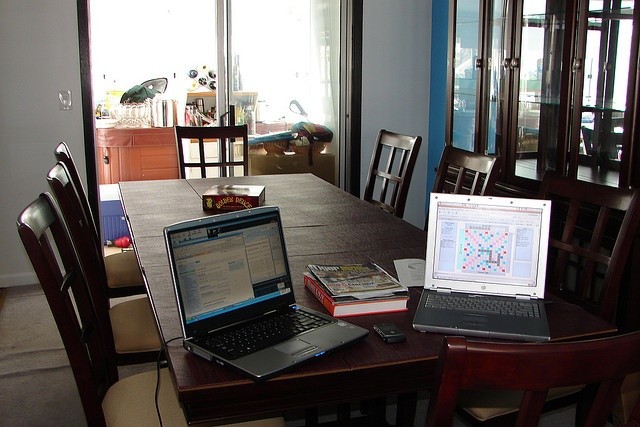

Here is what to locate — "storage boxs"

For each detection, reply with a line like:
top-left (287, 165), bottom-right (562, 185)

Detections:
top-left (100, 199), bottom-right (130, 244)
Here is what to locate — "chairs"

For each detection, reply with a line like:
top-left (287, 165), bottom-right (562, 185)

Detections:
top-left (47, 162), bottom-right (163, 362)
top-left (17, 191), bottom-right (287, 426)
top-left (54, 141), bottom-right (146, 298)
top-left (175, 124), bottom-right (248, 179)
top-left (451, 168), bottom-right (640, 423)
top-left (361, 130), bottom-right (422, 220)
top-left (431, 334), bottom-right (640, 426)
top-left (425, 143), bottom-right (497, 233)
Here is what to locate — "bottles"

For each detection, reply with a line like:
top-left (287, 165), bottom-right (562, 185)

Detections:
top-left (196, 98), bottom-right (204, 113)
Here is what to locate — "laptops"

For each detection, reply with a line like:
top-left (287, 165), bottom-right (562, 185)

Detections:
top-left (162, 205), bottom-right (370, 384)
top-left (409, 193), bottom-right (553, 343)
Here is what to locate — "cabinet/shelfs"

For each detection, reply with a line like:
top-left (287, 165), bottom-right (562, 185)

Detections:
top-left (182, 91), bottom-right (259, 179)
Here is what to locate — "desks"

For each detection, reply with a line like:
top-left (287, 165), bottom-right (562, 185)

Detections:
top-left (116, 173), bottom-right (617, 426)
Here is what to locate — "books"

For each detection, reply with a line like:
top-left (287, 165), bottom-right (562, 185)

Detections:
top-left (300, 259), bottom-right (410, 319)
